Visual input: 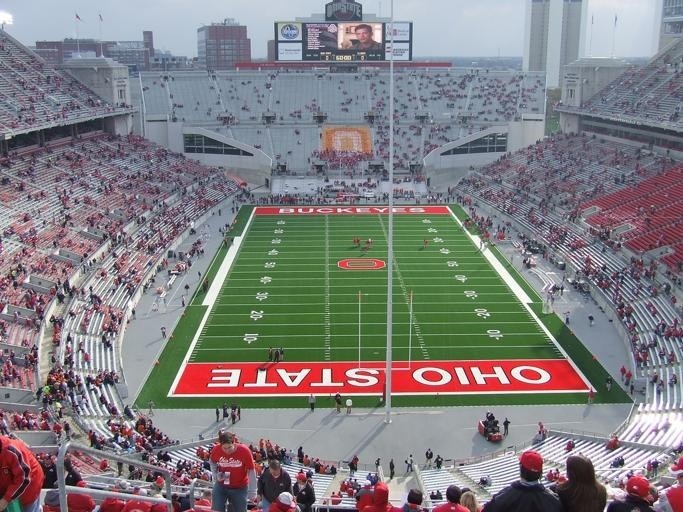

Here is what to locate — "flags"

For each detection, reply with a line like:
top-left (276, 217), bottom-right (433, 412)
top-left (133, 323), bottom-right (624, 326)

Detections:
top-left (75, 14), bottom-right (80, 22)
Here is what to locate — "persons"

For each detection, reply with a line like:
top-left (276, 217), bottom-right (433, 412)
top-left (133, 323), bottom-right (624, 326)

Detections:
top-left (348, 24), bottom-right (381, 51)
top-left (308, 393), bottom-right (315, 412)
top-left (345, 396), bottom-right (352, 415)
top-left (334, 391), bottom-right (341, 406)
top-left (245, 436), bottom-right (462, 512)
top-left (245, 53), bottom-right (682, 205)
top-left (279, 347), bottom-right (283, 361)
top-left (353, 236), bottom-right (372, 253)
top-left (336, 397), bottom-right (341, 413)
top-left (423, 239), bottom-right (427, 248)
top-left (268, 346), bottom-right (273, 362)
top-left (273, 349), bottom-right (279, 362)
top-left (0, 39), bottom-right (245, 512)
top-left (462, 205), bottom-right (682, 511)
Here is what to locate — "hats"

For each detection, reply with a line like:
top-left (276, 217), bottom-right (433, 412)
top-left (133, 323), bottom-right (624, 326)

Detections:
top-left (295, 471), bottom-right (308, 481)
top-left (407, 488), bottom-right (423, 502)
top-left (277, 491), bottom-right (296, 507)
top-left (626, 475), bottom-right (651, 496)
top-left (520, 451), bottom-right (544, 476)
top-left (668, 457), bottom-right (683, 474)
top-left (447, 485), bottom-right (461, 502)
